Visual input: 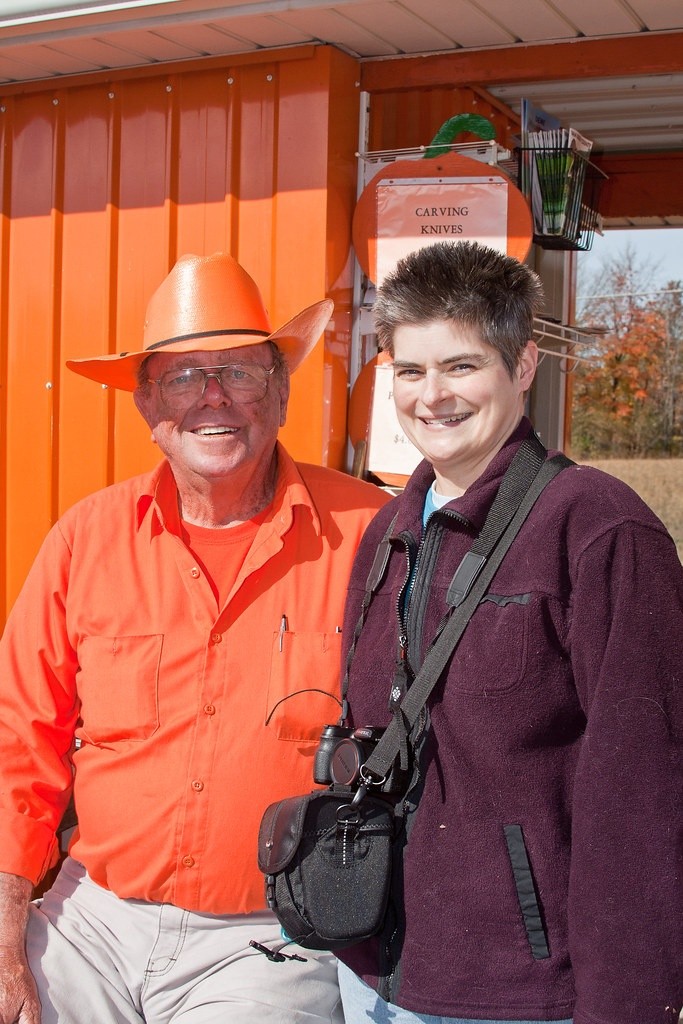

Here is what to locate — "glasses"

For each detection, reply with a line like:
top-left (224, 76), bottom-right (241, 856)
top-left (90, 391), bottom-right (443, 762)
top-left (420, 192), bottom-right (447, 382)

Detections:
top-left (146, 363), bottom-right (276, 410)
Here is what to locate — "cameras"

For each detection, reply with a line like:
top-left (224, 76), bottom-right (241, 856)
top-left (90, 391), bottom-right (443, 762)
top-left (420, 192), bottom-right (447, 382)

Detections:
top-left (312, 723), bottom-right (411, 800)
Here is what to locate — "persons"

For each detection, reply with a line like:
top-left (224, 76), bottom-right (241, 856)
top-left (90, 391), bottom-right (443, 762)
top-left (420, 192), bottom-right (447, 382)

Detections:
top-left (335, 240), bottom-right (683, 1024)
top-left (1, 253), bottom-right (399, 1024)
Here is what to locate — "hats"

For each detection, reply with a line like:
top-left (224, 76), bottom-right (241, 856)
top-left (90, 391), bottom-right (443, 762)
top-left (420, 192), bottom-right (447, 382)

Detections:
top-left (66, 250), bottom-right (334, 392)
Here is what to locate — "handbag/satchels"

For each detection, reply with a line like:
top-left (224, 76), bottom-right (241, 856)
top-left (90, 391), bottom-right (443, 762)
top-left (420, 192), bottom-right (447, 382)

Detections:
top-left (256, 792), bottom-right (395, 951)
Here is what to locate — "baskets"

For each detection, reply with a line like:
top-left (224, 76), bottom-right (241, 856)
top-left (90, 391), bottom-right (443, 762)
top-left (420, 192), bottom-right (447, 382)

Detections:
top-left (514, 147), bottom-right (610, 251)
